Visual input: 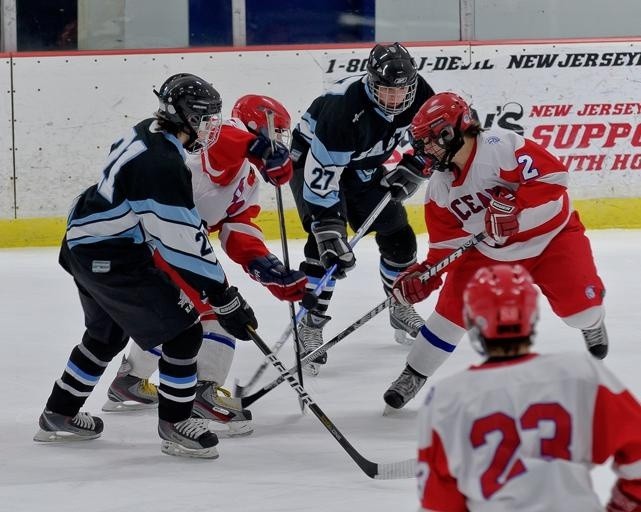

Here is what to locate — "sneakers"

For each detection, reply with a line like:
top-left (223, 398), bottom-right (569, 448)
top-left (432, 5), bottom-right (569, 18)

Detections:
top-left (296, 304), bottom-right (428, 409)
top-left (39, 354), bottom-right (253, 449)
top-left (582, 320), bottom-right (608, 359)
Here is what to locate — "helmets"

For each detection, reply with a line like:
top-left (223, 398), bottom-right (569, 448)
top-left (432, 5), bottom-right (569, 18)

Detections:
top-left (154, 72), bottom-right (294, 157)
top-left (410, 94), bottom-right (481, 173)
top-left (458, 264), bottom-right (540, 357)
top-left (366, 40), bottom-right (418, 117)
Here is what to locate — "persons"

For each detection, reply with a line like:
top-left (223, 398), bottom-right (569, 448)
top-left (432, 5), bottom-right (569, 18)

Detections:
top-left (379, 91), bottom-right (612, 407)
top-left (417, 263), bottom-right (639, 512)
top-left (290, 43), bottom-right (440, 369)
top-left (38, 72), bottom-right (262, 452)
top-left (106, 92), bottom-right (305, 434)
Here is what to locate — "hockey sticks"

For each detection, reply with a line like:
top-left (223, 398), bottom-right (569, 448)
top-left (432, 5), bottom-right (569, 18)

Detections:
top-left (211, 109), bottom-right (489, 479)
top-left (240, 230), bottom-right (490, 412)
top-left (243, 320), bottom-right (420, 478)
top-left (235, 189), bottom-right (393, 398)
top-left (264, 108), bottom-right (307, 414)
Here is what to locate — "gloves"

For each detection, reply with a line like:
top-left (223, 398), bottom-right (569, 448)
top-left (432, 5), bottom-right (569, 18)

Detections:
top-left (247, 253), bottom-right (309, 302)
top-left (379, 154), bottom-right (432, 204)
top-left (208, 285), bottom-right (258, 342)
top-left (310, 218), bottom-right (357, 279)
top-left (486, 185), bottom-right (526, 249)
top-left (246, 131), bottom-right (293, 187)
